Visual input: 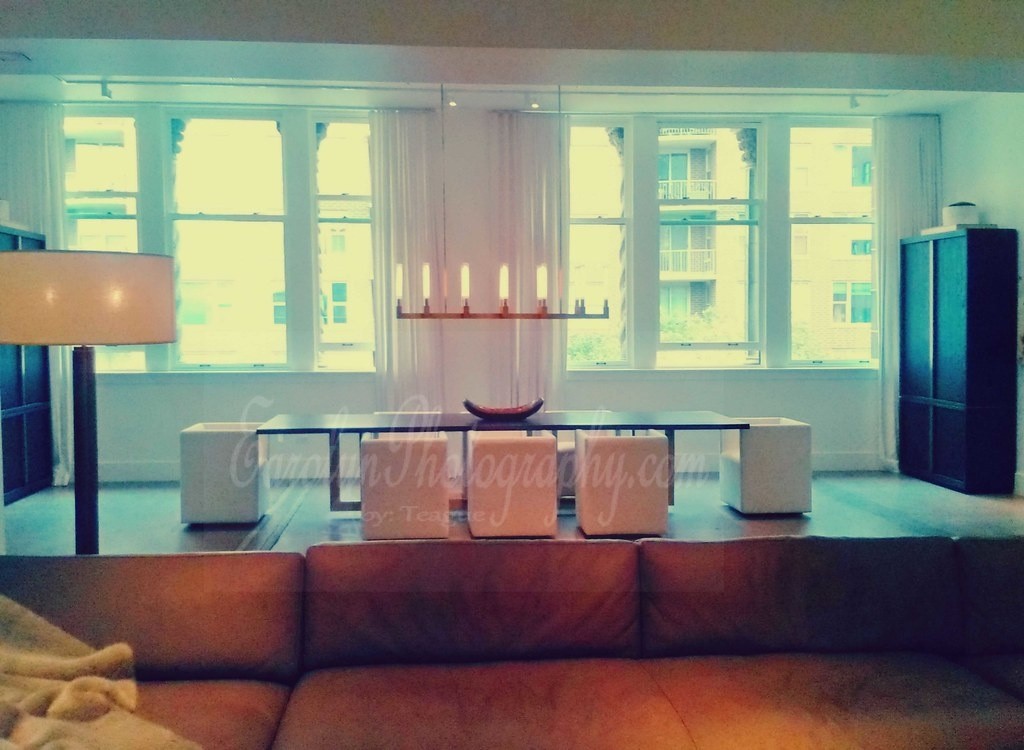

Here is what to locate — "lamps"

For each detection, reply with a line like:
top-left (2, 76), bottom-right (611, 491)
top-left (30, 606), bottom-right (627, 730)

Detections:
top-left (0, 249), bottom-right (176, 555)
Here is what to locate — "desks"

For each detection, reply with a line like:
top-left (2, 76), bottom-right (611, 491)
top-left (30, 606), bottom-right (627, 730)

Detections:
top-left (258, 412), bottom-right (752, 512)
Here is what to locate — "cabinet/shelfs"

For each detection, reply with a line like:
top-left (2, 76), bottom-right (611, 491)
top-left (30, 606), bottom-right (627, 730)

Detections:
top-left (0, 225), bottom-right (54, 506)
top-left (897, 229), bottom-right (1019, 495)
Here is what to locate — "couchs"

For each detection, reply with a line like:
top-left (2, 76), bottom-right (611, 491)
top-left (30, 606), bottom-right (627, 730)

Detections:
top-left (370, 411), bottom-right (466, 511)
top-left (466, 430), bottom-right (558, 538)
top-left (719, 417), bottom-right (817, 517)
top-left (575, 429), bottom-right (668, 537)
top-left (0, 536), bottom-right (1024, 750)
top-left (541, 410), bottom-right (618, 496)
top-left (179, 421), bottom-right (272, 525)
top-left (360, 432), bottom-right (448, 539)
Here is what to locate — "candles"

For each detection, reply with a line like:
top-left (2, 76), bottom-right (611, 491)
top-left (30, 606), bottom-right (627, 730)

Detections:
top-left (461, 262), bottom-right (470, 299)
top-left (395, 263), bottom-right (402, 299)
top-left (422, 264), bottom-right (430, 298)
top-left (536, 265), bottom-right (548, 299)
top-left (499, 264), bottom-right (509, 299)
